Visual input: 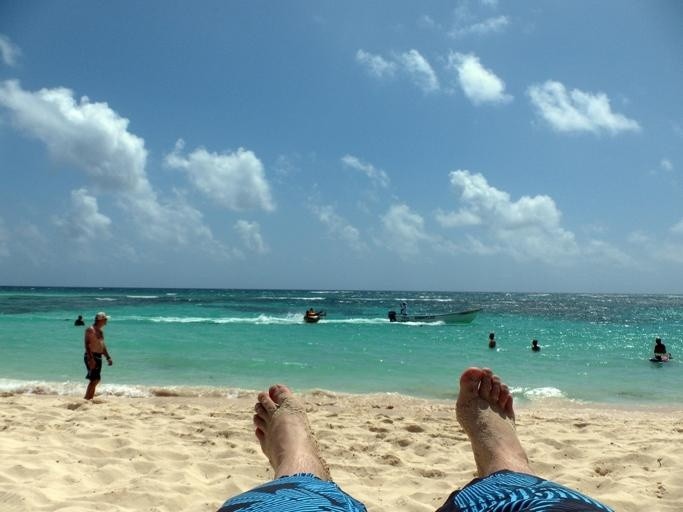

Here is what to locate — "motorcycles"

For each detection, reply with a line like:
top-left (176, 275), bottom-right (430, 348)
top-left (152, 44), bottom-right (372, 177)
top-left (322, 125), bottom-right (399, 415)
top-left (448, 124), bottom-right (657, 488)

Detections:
top-left (303, 312), bottom-right (320, 323)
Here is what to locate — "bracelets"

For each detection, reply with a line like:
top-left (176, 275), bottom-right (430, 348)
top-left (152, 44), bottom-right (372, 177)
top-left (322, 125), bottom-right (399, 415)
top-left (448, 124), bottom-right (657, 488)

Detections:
top-left (105, 356), bottom-right (110, 360)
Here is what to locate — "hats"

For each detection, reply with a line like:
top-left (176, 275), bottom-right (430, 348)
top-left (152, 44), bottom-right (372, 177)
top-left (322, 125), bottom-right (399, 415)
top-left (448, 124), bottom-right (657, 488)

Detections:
top-left (96, 313), bottom-right (111, 319)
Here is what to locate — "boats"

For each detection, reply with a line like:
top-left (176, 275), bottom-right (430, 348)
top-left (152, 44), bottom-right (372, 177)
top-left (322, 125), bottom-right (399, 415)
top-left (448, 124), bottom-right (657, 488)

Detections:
top-left (387, 307), bottom-right (484, 325)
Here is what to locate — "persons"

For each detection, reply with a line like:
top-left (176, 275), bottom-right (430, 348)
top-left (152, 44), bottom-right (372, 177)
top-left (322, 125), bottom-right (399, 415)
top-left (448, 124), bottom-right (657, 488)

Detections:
top-left (399, 301), bottom-right (403, 313)
top-left (489, 333), bottom-right (496, 348)
top-left (401, 302), bottom-right (407, 314)
top-left (74, 315), bottom-right (84, 325)
top-left (215, 365), bottom-right (610, 512)
top-left (82, 311), bottom-right (113, 399)
top-left (305, 308), bottom-right (325, 316)
top-left (654, 338), bottom-right (666, 354)
top-left (650, 353), bottom-right (673, 362)
top-left (531, 340), bottom-right (540, 351)
